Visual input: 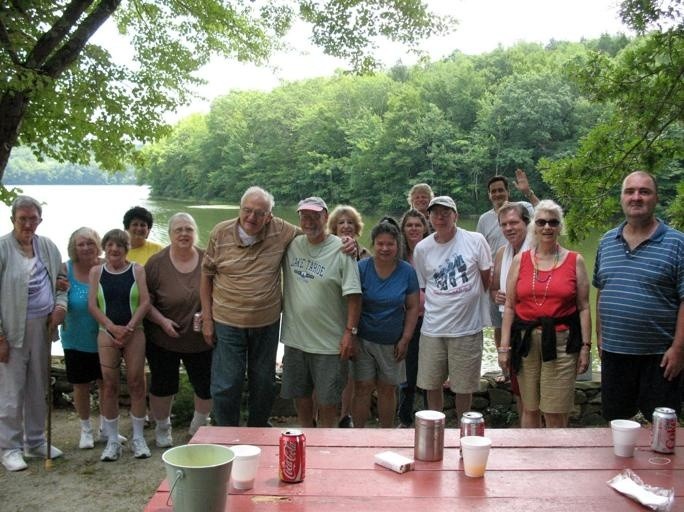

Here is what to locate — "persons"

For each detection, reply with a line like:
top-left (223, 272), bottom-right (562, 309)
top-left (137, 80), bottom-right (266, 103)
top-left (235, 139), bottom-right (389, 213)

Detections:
top-left (0, 168), bottom-right (593, 472)
top-left (592, 170), bottom-right (683, 428)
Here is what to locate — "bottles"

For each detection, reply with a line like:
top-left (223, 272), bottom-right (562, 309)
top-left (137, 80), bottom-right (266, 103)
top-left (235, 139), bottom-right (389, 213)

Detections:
top-left (415, 410), bottom-right (446, 462)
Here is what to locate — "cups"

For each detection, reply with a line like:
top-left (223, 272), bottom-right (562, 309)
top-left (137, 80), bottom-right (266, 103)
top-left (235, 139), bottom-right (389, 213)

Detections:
top-left (230, 445), bottom-right (261, 490)
top-left (609, 418), bottom-right (640, 457)
top-left (459, 437), bottom-right (491, 478)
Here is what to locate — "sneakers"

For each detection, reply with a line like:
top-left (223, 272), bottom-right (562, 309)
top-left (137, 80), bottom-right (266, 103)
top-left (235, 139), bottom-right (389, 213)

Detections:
top-left (188, 421), bottom-right (206, 437)
top-left (24, 442), bottom-right (62, 459)
top-left (100, 441), bottom-right (122, 461)
top-left (99, 429), bottom-right (127, 444)
top-left (0, 449), bottom-right (28, 471)
top-left (79, 428), bottom-right (94, 449)
top-left (130, 437), bottom-right (151, 458)
top-left (155, 423), bottom-right (173, 448)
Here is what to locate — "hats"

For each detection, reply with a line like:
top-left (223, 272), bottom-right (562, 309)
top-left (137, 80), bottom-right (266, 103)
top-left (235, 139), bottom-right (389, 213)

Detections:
top-left (295, 197), bottom-right (329, 214)
top-left (426, 195), bottom-right (457, 215)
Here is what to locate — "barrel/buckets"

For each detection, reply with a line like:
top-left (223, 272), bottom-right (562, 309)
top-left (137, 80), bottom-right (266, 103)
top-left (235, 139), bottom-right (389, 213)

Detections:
top-left (161, 443), bottom-right (236, 512)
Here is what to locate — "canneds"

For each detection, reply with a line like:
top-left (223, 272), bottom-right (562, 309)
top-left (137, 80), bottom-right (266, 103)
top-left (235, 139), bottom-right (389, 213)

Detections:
top-left (192, 311), bottom-right (202, 333)
top-left (649, 407), bottom-right (677, 454)
top-left (459, 411), bottom-right (485, 457)
top-left (279, 430), bottom-right (306, 483)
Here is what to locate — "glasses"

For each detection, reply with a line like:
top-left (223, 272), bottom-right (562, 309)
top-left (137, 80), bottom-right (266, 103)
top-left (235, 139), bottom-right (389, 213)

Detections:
top-left (241, 208), bottom-right (269, 218)
top-left (535, 219), bottom-right (560, 228)
top-left (19, 215), bottom-right (39, 226)
top-left (297, 213), bottom-right (321, 220)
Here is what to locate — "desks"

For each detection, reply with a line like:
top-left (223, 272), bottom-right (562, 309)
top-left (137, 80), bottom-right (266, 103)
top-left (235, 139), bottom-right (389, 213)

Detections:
top-left (141, 426), bottom-right (684, 512)
